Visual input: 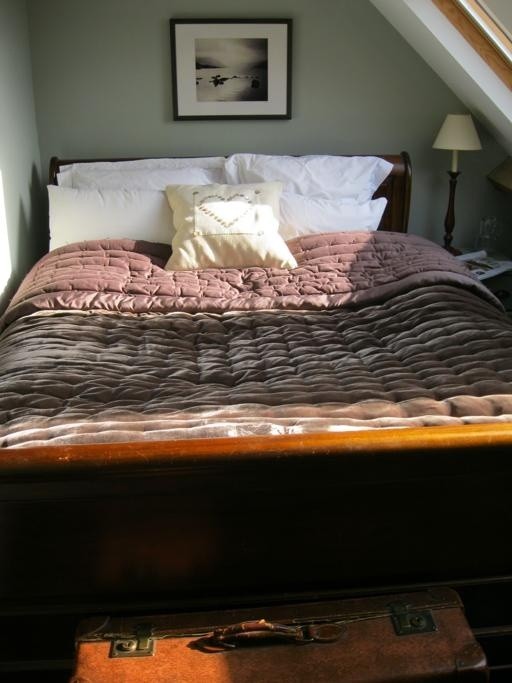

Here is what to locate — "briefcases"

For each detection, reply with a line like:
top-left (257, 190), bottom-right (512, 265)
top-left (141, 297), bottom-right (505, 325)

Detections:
top-left (74, 585), bottom-right (490, 683)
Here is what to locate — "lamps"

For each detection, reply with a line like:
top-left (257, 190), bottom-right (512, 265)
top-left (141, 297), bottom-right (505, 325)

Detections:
top-left (432, 114), bottom-right (483, 254)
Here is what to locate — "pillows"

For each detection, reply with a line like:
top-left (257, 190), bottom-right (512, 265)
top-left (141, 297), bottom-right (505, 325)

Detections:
top-left (226, 155), bottom-right (393, 196)
top-left (279, 194), bottom-right (391, 246)
top-left (166, 179), bottom-right (300, 270)
top-left (54, 157), bottom-right (228, 187)
top-left (46, 181), bottom-right (180, 251)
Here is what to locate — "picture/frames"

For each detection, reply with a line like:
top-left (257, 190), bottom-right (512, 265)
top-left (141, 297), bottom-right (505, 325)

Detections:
top-left (170, 16), bottom-right (293, 122)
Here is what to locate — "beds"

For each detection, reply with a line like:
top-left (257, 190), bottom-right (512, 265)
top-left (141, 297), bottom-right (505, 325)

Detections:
top-left (0, 150), bottom-right (511, 613)
